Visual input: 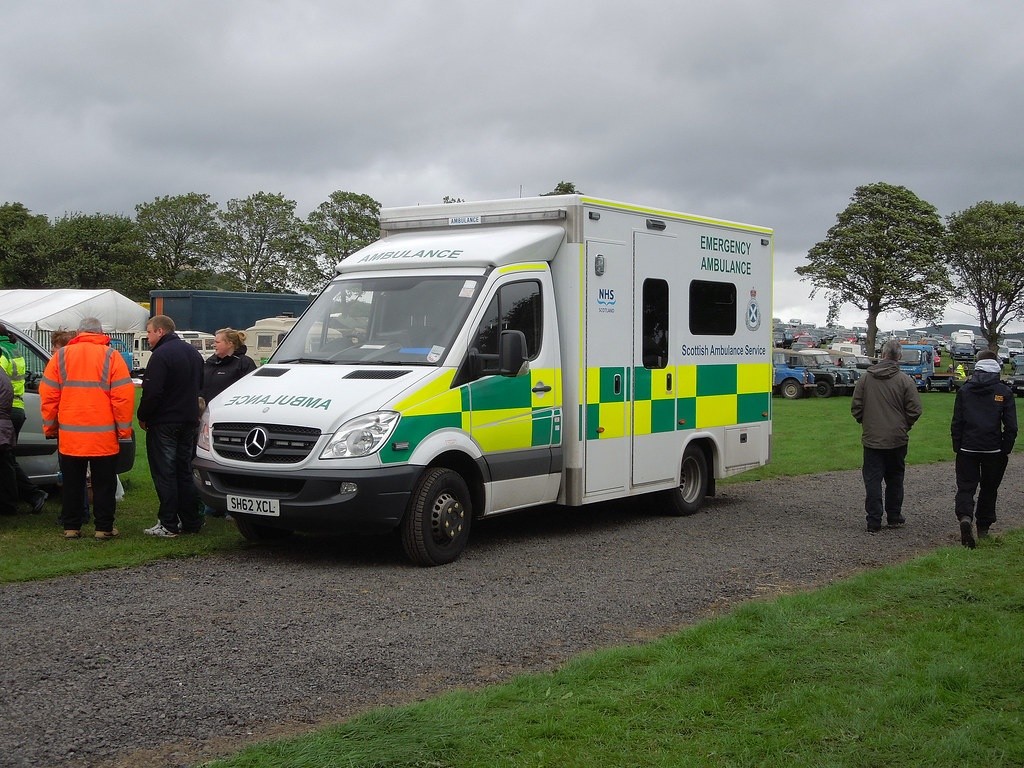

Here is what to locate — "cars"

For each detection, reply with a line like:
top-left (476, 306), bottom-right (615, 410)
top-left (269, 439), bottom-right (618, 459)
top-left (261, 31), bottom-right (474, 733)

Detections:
top-left (772, 316), bottom-right (1024, 401)
top-left (0, 316), bottom-right (137, 494)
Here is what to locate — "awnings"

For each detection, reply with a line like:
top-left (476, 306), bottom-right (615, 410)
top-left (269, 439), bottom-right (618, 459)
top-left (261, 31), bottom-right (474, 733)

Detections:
top-left (0, 289), bottom-right (150, 333)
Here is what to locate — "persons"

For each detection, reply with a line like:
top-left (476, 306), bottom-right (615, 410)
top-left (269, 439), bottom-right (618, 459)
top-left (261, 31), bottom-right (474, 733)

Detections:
top-left (947, 364), bottom-right (953, 373)
top-left (137, 315), bottom-right (205, 537)
top-left (950, 351), bottom-right (1017, 548)
top-left (203, 328), bottom-right (257, 521)
top-left (0, 324), bottom-right (48, 512)
top-left (956, 362), bottom-right (966, 379)
top-left (51, 333), bottom-right (125, 502)
top-left (39, 317), bottom-right (135, 539)
top-left (0, 368), bottom-right (18, 516)
top-left (851, 342), bottom-right (923, 531)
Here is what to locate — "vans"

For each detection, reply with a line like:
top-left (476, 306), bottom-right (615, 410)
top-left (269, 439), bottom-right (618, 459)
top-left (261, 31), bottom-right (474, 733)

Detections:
top-left (132, 329), bottom-right (218, 380)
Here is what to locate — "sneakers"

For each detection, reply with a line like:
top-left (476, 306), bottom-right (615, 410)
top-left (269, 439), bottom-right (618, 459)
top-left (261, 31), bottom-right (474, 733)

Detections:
top-left (63, 528), bottom-right (81, 538)
top-left (145, 519), bottom-right (180, 537)
top-left (92, 527), bottom-right (119, 540)
top-left (33, 490), bottom-right (49, 514)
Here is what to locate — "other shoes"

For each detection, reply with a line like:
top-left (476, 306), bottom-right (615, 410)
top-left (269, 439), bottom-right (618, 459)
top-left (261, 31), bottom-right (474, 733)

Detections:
top-left (978, 529), bottom-right (989, 539)
top-left (886, 518), bottom-right (907, 528)
top-left (959, 515), bottom-right (976, 550)
top-left (867, 523), bottom-right (878, 534)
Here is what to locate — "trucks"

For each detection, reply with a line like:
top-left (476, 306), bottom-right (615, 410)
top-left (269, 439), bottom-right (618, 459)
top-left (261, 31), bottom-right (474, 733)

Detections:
top-left (240, 315), bottom-right (343, 370)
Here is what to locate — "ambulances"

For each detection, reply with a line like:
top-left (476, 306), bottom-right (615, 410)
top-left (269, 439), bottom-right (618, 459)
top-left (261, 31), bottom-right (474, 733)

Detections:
top-left (187, 193), bottom-right (778, 569)
